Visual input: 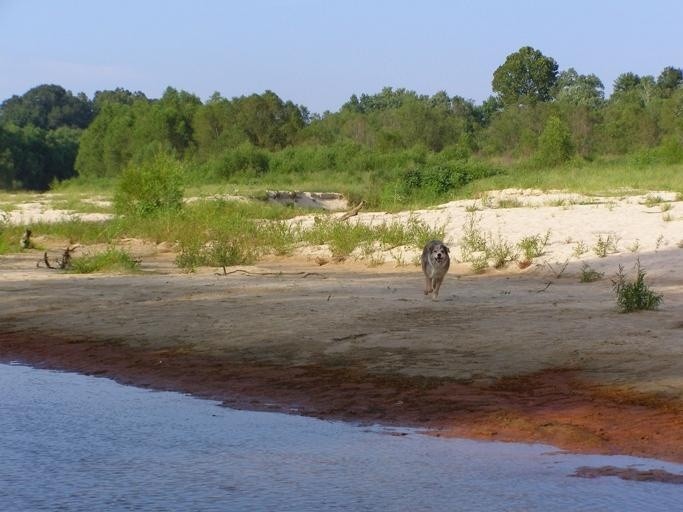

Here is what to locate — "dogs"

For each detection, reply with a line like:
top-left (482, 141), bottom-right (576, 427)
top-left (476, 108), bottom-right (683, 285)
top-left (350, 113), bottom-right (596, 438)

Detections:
top-left (421, 239), bottom-right (451, 301)
